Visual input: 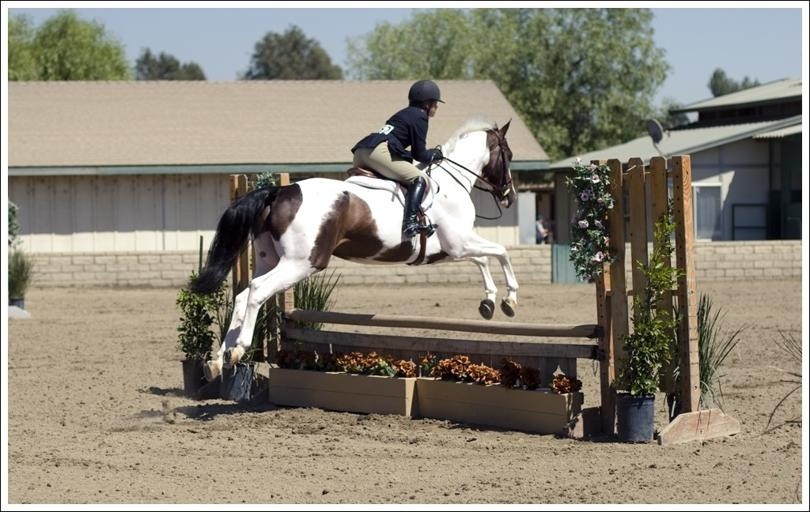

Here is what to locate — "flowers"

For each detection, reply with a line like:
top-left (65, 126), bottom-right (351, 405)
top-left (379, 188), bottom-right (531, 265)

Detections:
top-left (416, 352), bottom-right (585, 394)
top-left (269, 347), bottom-right (425, 376)
top-left (564, 157), bottom-right (620, 282)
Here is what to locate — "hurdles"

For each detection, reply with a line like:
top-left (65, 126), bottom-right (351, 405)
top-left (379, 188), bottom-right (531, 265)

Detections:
top-left (229, 155), bottom-right (740, 446)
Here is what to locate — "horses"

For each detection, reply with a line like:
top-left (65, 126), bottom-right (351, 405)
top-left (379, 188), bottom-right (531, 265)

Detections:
top-left (190, 117), bottom-right (520, 383)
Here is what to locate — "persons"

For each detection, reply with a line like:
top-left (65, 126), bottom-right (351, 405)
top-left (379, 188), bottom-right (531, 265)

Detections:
top-left (351, 81), bottom-right (444, 237)
top-left (537, 214), bottom-right (549, 244)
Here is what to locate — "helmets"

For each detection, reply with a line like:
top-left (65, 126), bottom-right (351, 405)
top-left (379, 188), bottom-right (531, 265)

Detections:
top-left (408, 80), bottom-right (446, 106)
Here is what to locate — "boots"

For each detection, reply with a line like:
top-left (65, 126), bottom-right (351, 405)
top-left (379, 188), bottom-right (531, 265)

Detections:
top-left (402, 176), bottom-right (430, 237)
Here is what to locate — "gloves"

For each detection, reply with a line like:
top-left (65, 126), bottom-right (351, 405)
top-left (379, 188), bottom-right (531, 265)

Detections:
top-left (429, 148), bottom-right (443, 163)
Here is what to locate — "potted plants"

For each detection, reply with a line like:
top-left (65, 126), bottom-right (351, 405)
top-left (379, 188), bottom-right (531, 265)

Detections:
top-left (173, 262), bottom-right (232, 398)
top-left (614, 198), bottom-right (687, 444)
